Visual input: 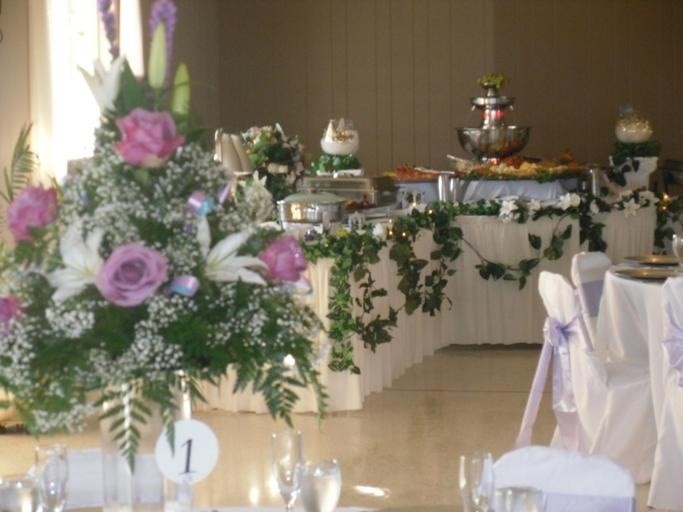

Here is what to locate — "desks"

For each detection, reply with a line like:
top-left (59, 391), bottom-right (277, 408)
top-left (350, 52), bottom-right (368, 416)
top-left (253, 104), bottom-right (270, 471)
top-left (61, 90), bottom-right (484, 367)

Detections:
top-left (189, 162), bottom-right (683, 414)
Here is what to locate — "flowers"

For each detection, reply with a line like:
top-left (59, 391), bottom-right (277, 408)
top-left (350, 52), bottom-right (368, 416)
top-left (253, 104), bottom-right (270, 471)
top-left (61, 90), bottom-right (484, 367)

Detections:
top-left (241, 123), bottom-right (305, 205)
top-left (1, 56), bottom-right (330, 476)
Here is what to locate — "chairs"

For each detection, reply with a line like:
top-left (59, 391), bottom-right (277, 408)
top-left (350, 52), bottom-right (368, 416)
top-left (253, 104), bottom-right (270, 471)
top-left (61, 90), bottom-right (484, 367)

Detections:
top-left (522, 247), bottom-right (683, 510)
top-left (475, 441), bottom-right (636, 510)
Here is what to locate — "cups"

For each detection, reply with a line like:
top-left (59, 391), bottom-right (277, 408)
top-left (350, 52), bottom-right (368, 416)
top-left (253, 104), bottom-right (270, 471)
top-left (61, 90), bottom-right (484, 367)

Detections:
top-left (0, 443), bottom-right (69, 511)
top-left (438, 174), bottom-right (460, 200)
top-left (460, 451), bottom-right (545, 512)
top-left (270, 431), bottom-right (342, 511)
top-left (672, 233), bottom-right (683, 263)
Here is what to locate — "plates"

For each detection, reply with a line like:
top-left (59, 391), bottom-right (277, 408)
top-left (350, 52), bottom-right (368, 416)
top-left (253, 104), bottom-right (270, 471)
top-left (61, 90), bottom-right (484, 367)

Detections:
top-left (613, 252), bottom-right (679, 280)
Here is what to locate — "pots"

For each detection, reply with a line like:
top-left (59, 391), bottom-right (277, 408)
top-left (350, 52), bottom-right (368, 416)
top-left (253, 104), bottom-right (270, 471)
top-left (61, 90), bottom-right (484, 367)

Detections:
top-left (276, 188), bottom-right (347, 231)
